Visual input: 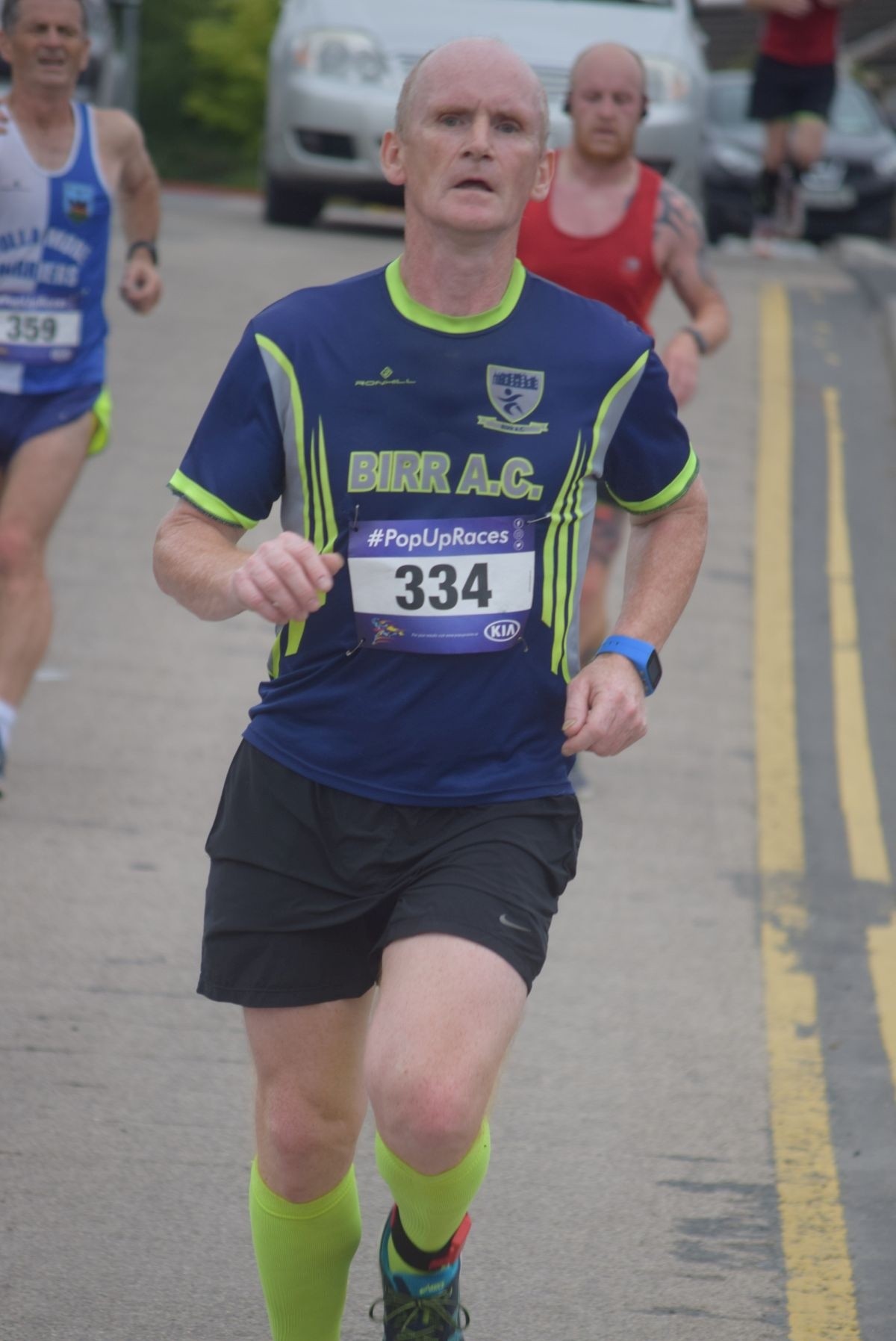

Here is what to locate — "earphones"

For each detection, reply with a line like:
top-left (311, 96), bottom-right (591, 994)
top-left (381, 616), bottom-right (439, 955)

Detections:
top-left (562, 103), bottom-right (570, 112)
top-left (642, 109), bottom-right (647, 118)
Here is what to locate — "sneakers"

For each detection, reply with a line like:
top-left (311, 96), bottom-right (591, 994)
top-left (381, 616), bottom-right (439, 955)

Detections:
top-left (369, 1203), bottom-right (470, 1341)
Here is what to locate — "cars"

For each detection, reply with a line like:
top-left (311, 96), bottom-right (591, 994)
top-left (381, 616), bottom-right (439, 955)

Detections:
top-left (705, 65), bottom-right (895, 240)
top-left (257, 0), bottom-right (722, 231)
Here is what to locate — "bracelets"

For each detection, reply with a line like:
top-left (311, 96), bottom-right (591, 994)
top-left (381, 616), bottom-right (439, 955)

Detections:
top-left (680, 324), bottom-right (710, 361)
top-left (126, 239), bottom-right (158, 268)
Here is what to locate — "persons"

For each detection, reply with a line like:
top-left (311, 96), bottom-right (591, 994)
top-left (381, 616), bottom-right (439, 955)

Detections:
top-left (742, 0), bottom-right (840, 246)
top-left (152, 36), bottom-right (712, 1340)
top-left (514, 42), bottom-right (733, 672)
top-left (0, 1), bottom-right (164, 802)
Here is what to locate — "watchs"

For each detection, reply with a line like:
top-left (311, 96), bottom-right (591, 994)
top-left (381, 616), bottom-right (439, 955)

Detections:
top-left (593, 637), bottom-right (662, 699)
